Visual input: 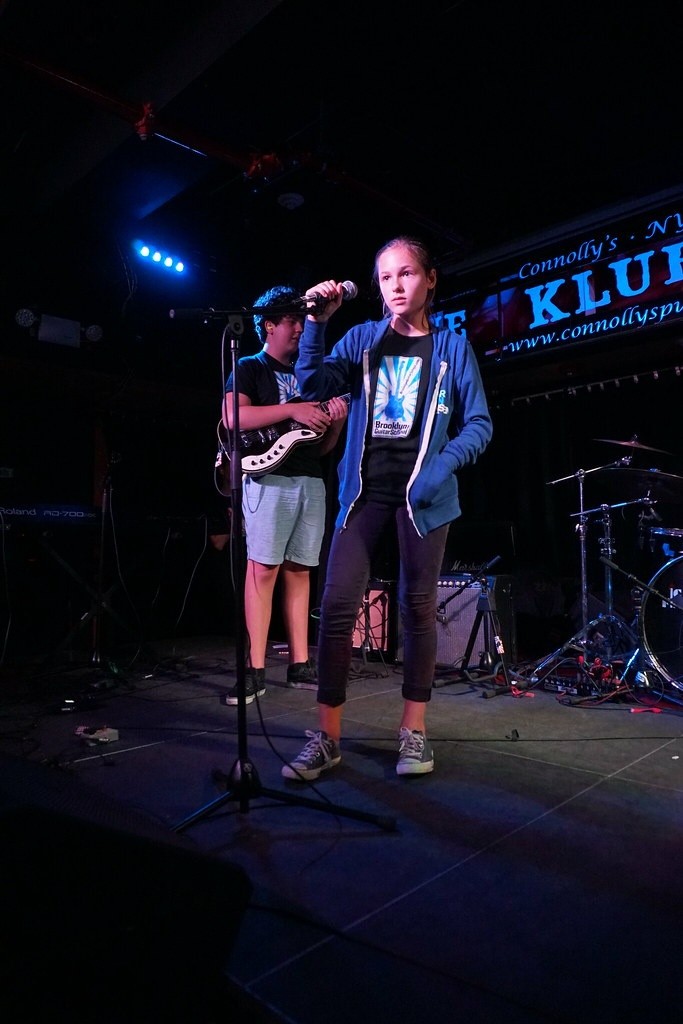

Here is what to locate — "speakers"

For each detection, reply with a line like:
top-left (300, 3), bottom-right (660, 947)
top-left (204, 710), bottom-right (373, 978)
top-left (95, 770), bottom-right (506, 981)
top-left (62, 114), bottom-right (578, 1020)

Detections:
top-left (397, 575), bottom-right (517, 672)
top-left (349, 583), bottom-right (402, 660)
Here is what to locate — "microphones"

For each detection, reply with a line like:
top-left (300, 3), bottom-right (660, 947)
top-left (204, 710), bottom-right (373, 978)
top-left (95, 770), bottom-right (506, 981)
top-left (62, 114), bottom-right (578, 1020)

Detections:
top-left (95, 451), bottom-right (119, 488)
top-left (292, 280), bottom-right (357, 307)
top-left (436, 602), bottom-right (445, 612)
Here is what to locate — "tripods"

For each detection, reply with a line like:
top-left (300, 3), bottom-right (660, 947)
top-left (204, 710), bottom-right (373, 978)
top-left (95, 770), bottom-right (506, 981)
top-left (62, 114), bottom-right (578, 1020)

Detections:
top-left (167, 298), bottom-right (397, 836)
top-left (440, 556), bottom-right (502, 677)
top-left (526, 447), bottom-right (683, 708)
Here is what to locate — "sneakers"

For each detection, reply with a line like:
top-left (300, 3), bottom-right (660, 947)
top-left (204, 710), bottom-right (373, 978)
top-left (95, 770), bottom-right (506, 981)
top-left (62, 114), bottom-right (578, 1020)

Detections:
top-left (396, 726), bottom-right (434, 775)
top-left (286, 660), bottom-right (320, 691)
top-left (226, 667), bottom-right (266, 705)
top-left (281, 729), bottom-right (342, 781)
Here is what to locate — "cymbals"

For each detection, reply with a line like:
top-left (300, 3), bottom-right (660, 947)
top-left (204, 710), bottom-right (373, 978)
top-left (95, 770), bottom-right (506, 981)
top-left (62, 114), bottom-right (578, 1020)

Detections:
top-left (604, 467), bottom-right (683, 490)
top-left (590, 438), bottom-right (675, 457)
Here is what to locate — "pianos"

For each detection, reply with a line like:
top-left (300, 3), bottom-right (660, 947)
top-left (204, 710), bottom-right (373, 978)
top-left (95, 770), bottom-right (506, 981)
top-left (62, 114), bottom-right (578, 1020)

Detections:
top-left (0, 502), bottom-right (102, 531)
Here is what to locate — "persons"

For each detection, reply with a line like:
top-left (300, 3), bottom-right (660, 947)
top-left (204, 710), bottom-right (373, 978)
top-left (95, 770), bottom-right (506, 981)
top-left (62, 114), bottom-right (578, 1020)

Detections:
top-left (281, 237), bottom-right (495, 781)
top-left (223, 285), bottom-right (348, 704)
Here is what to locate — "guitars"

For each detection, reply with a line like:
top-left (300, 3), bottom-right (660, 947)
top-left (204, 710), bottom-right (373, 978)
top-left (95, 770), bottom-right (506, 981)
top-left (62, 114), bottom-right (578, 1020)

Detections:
top-left (216, 392), bottom-right (351, 478)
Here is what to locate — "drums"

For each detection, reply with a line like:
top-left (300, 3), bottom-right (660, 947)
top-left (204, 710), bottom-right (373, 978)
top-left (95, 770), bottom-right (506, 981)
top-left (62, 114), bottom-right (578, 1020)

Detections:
top-left (642, 525), bottom-right (683, 565)
top-left (637, 556), bottom-right (683, 692)
